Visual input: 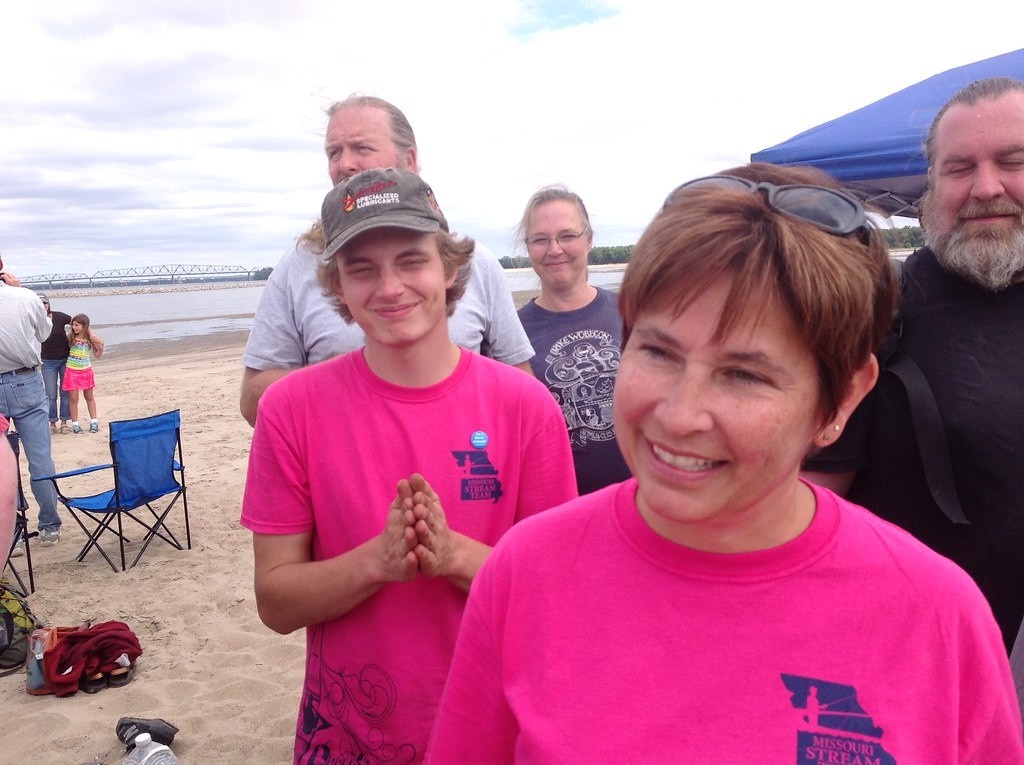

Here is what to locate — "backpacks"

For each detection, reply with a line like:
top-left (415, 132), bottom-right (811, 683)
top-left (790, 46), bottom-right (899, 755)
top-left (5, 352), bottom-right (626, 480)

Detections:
top-left (0, 575), bottom-right (44, 677)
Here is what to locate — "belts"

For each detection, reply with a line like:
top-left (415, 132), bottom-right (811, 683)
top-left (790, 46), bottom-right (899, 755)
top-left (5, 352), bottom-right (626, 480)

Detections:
top-left (0, 366), bottom-right (35, 376)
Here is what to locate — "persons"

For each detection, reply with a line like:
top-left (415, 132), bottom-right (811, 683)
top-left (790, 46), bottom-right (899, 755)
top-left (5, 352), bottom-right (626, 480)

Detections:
top-left (507, 186), bottom-right (655, 494)
top-left (416, 159), bottom-right (1023, 765)
top-left (785, 78), bottom-right (1024, 654)
top-left (62, 315), bottom-right (105, 435)
top-left (0, 253), bottom-right (61, 557)
top-left (240, 163), bottom-right (576, 765)
top-left (239, 96), bottom-right (541, 428)
top-left (35, 293), bottom-right (104, 434)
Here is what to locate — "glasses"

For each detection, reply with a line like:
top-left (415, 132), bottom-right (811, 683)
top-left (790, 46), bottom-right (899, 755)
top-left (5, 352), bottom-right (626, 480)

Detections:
top-left (523, 225), bottom-right (587, 248)
top-left (43, 301), bottom-right (48, 305)
top-left (657, 175), bottom-right (876, 254)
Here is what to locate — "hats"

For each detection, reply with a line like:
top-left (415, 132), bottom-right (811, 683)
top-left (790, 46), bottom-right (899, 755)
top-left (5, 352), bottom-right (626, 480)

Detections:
top-left (37, 292), bottom-right (49, 303)
top-left (320, 170), bottom-right (449, 269)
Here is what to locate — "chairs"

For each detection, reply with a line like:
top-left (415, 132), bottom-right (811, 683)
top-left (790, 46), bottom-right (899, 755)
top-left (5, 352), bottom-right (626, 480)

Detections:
top-left (31, 406), bottom-right (195, 573)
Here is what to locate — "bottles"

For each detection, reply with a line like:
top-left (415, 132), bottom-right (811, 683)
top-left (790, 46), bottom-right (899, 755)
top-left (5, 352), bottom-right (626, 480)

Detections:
top-left (120, 731), bottom-right (178, 764)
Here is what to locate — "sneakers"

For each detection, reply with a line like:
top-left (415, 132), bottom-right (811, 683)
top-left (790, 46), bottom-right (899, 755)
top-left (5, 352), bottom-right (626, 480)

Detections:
top-left (39, 528), bottom-right (61, 548)
top-left (10, 529), bottom-right (24, 557)
top-left (49, 426), bottom-right (57, 436)
top-left (82, 663), bottom-right (136, 695)
top-left (59, 424), bottom-right (70, 435)
top-left (73, 425), bottom-right (83, 434)
top-left (114, 713), bottom-right (179, 749)
top-left (89, 421), bottom-right (98, 432)
